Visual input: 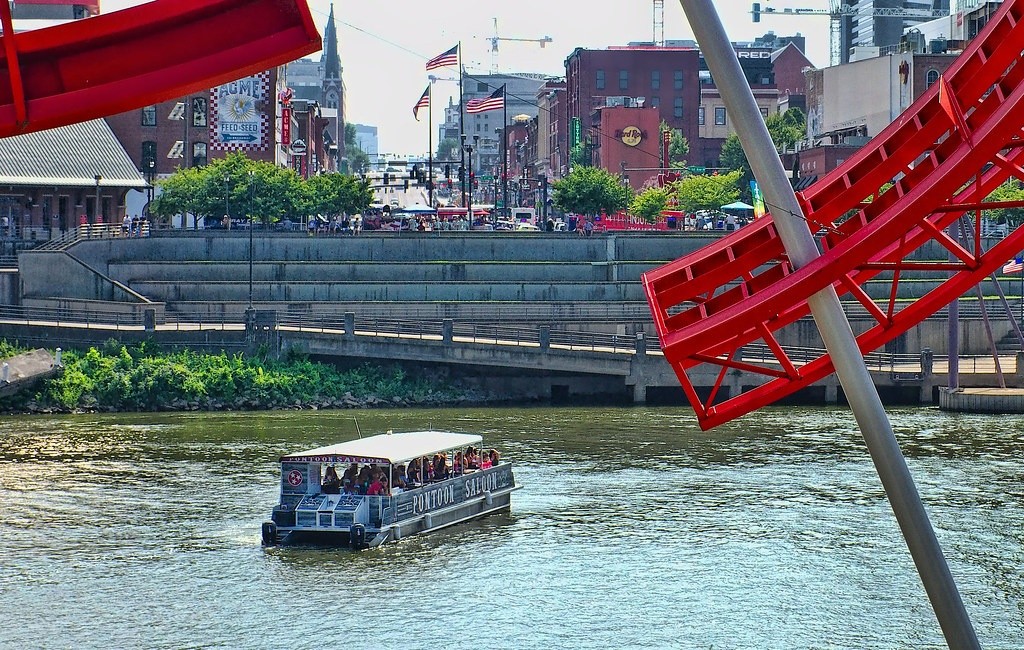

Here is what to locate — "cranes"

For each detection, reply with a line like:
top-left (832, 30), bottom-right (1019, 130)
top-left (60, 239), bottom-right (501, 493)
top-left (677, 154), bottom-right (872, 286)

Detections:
top-left (653, 0), bottom-right (665, 48)
top-left (485, 18), bottom-right (554, 75)
top-left (748, 0), bottom-right (949, 67)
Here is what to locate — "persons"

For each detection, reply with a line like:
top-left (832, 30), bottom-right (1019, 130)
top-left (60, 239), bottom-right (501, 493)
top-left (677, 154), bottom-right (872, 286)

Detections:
top-left (407, 453), bottom-right (451, 483)
top-left (547, 219), bottom-right (554, 231)
top-left (401, 215), bottom-right (491, 230)
top-left (283, 217), bottom-right (292, 230)
top-left (576, 220), bottom-right (594, 236)
top-left (222, 215), bottom-right (229, 228)
top-left (322, 464), bottom-right (388, 495)
top-left (393, 465), bottom-right (409, 487)
top-left (724, 213), bottom-right (735, 224)
top-left (308, 217), bottom-right (361, 236)
top-left (454, 447), bottom-right (500, 474)
top-left (122, 215), bottom-right (146, 237)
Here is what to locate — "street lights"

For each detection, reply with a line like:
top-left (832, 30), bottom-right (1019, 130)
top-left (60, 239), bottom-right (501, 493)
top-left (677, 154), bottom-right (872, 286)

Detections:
top-left (623, 174), bottom-right (630, 231)
top-left (223, 172), bottom-right (230, 216)
top-left (493, 175), bottom-right (499, 230)
top-left (460, 133), bottom-right (480, 229)
top-left (149, 154), bottom-right (155, 200)
top-left (94, 174), bottom-right (101, 224)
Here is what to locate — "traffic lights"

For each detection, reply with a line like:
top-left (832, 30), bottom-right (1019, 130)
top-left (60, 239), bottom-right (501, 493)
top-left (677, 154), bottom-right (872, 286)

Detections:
top-left (448, 179), bottom-right (452, 189)
top-left (471, 172), bottom-right (474, 183)
top-left (474, 180), bottom-right (479, 190)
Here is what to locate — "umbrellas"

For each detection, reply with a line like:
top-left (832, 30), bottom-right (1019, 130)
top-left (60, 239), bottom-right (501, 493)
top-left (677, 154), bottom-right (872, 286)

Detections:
top-left (721, 202), bottom-right (754, 211)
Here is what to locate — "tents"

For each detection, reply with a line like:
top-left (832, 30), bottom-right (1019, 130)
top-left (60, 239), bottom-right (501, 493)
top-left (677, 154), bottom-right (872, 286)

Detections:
top-left (402, 204), bottom-right (437, 214)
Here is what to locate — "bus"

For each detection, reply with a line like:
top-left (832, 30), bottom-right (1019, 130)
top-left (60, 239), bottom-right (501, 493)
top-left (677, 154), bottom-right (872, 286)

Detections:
top-left (437, 207), bottom-right (494, 229)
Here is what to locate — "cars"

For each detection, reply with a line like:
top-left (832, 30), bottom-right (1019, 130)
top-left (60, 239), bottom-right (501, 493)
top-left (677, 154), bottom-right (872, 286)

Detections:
top-left (493, 216), bottom-right (542, 233)
top-left (390, 197), bottom-right (399, 206)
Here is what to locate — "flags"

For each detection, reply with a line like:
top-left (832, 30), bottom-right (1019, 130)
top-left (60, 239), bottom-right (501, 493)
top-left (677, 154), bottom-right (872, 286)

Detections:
top-left (467, 86), bottom-right (504, 113)
top-left (1003, 257), bottom-right (1023, 273)
top-left (426, 44), bottom-right (458, 70)
top-left (413, 86), bottom-right (430, 121)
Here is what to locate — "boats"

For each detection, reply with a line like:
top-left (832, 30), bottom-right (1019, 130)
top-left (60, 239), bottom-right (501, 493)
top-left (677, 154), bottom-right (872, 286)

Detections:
top-left (260, 423), bottom-right (524, 554)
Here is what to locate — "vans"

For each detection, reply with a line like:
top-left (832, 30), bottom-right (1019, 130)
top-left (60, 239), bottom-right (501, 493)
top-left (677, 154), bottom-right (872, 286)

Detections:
top-left (511, 207), bottom-right (537, 226)
